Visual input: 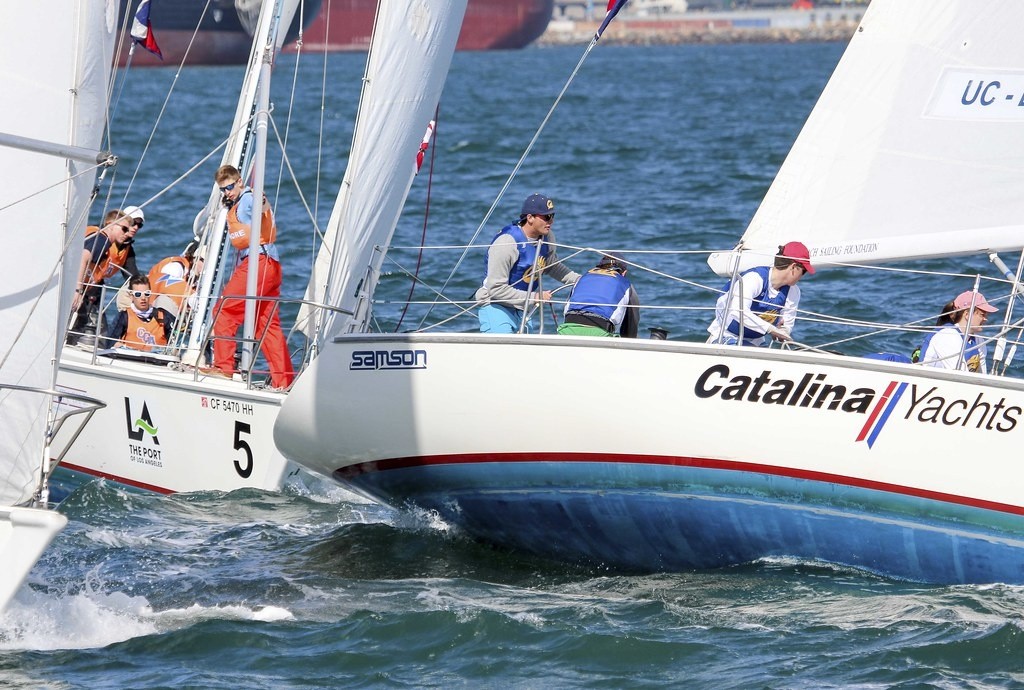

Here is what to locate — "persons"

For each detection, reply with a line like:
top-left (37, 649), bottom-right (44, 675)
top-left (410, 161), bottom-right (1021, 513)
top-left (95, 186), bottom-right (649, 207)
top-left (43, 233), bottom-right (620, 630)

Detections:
top-left (557, 253), bottom-right (640, 338)
top-left (918, 291), bottom-right (1000, 374)
top-left (197, 165), bottom-right (295, 392)
top-left (72, 205), bottom-right (207, 353)
top-left (706, 243), bottom-right (816, 348)
top-left (475, 194), bottom-right (582, 335)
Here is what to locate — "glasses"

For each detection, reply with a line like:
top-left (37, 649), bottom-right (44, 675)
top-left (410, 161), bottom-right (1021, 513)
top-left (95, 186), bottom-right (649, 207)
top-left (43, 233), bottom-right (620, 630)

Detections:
top-left (536, 213), bottom-right (555, 221)
top-left (129, 290), bottom-right (152, 299)
top-left (795, 264), bottom-right (808, 276)
top-left (219, 180), bottom-right (238, 192)
top-left (975, 309), bottom-right (987, 319)
top-left (132, 220), bottom-right (144, 229)
top-left (113, 221), bottom-right (129, 234)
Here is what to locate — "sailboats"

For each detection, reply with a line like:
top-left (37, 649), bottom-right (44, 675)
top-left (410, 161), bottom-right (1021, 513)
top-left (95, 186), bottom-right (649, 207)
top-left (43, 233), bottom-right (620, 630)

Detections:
top-left (271, 0), bottom-right (1024, 589)
top-left (0, 0), bottom-right (122, 615)
top-left (0, 0), bottom-right (468, 499)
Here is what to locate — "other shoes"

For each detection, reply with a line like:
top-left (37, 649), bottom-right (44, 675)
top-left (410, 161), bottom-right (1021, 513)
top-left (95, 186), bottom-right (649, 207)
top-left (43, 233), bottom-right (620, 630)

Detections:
top-left (189, 364), bottom-right (234, 380)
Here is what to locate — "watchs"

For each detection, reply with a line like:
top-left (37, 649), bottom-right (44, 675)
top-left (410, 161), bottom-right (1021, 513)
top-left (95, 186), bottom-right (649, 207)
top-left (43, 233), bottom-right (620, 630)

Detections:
top-left (76, 288), bottom-right (84, 294)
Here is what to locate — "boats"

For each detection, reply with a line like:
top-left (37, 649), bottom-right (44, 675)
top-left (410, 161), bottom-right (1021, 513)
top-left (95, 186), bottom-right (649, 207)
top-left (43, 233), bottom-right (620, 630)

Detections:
top-left (112, 0), bottom-right (553, 68)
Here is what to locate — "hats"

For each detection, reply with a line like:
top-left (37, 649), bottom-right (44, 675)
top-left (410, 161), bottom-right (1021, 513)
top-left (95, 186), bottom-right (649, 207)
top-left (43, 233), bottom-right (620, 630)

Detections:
top-left (779, 241), bottom-right (815, 275)
top-left (521, 193), bottom-right (557, 216)
top-left (953, 291), bottom-right (1000, 313)
top-left (599, 252), bottom-right (627, 269)
top-left (123, 205), bottom-right (145, 222)
